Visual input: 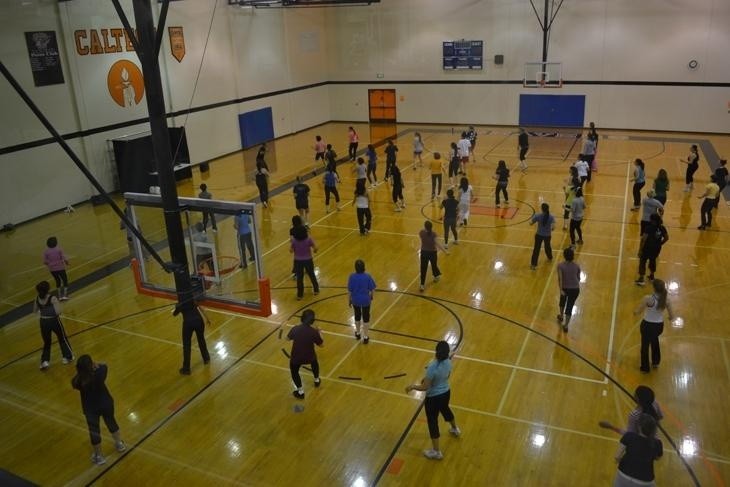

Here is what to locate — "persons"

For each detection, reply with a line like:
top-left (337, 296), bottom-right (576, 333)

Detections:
top-left (198, 183), bottom-right (218, 233)
top-left (232, 212), bottom-right (256, 267)
top-left (33, 280), bottom-right (75, 369)
top-left (255, 169), bottom-right (269, 207)
top-left (598, 384), bottom-right (663, 442)
top-left (171, 300), bottom-right (212, 375)
top-left (289, 224), bottom-right (319, 301)
top-left (555, 247), bottom-right (581, 333)
top-left (405, 340), bottom-right (462, 460)
top-left (292, 175), bottom-right (310, 224)
top-left (284, 308), bottom-right (325, 399)
top-left (290, 214), bottom-right (306, 279)
top-left (560, 121), bottom-right (729, 284)
top-left (256, 142), bottom-right (270, 174)
top-left (613, 413), bottom-right (664, 486)
top-left (347, 259), bottom-right (376, 344)
top-left (491, 160), bottom-right (510, 208)
top-left (418, 221), bottom-right (451, 291)
top-left (517, 128), bottom-right (529, 170)
top-left (310, 125), bottom-right (478, 250)
top-left (631, 278), bottom-right (674, 372)
top-left (43, 236), bottom-right (72, 301)
top-left (70, 354), bottom-right (128, 465)
top-left (529, 203), bottom-right (557, 269)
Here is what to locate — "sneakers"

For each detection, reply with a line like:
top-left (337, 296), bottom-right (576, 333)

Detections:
top-left (355, 331), bottom-right (369, 344)
top-left (90, 440), bottom-right (126, 465)
top-left (563, 166), bottom-right (596, 248)
top-left (424, 448), bottom-right (443, 459)
top-left (290, 263), bottom-right (320, 301)
top-left (198, 227), bottom-right (255, 277)
top-left (293, 378), bottom-right (320, 399)
top-left (180, 360), bottom-right (210, 375)
top-left (531, 255), bottom-right (552, 270)
top-left (641, 362), bottom-right (658, 374)
top-left (449, 427), bottom-right (461, 435)
top-left (557, 315), bottom-right (568, 333)
top-left (420, 239), bottom-right (458, 291)
top-left (635, 274), bottom-right (654, 285)
top-left (263, 159), bottom-right (527, 236)
top-left (58, 296), bottom-right (72, 301)
top-left (39, 355), bottom-right (76, 371)
top-left (631, 181), bottom-right (719, 230)
